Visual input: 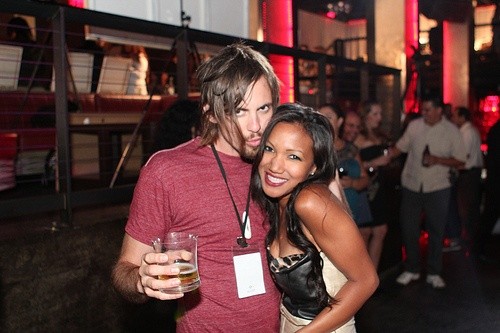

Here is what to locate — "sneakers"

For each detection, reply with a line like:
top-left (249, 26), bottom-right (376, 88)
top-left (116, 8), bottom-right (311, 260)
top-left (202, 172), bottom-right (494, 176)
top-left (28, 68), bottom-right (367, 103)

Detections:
top-left (396, 271), bottom-right (421, 285)
top-left (426, 274), bottom-right (445, 288)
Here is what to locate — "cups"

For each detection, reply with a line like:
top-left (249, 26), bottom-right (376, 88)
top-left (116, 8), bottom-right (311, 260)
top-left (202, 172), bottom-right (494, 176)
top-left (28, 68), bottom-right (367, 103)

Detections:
top-left (153, 233), bottom-right (200, 293)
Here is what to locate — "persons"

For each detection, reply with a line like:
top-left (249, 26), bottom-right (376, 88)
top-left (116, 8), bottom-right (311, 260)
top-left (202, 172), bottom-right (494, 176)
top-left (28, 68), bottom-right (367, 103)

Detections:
top-left (453, 106), bottom-right (483, 256)
top-left (384, 92), bottom-right (467, 288)
top-left (317, 99), bottom-right (421, 271)
top-left (251, 103), bottom-right (380, 333)
top-left (299, 41), bottom-right (336, 94)
top-left (111, 41), bottom-right (342, 333)
top-left (443, 104), bottom-right (453, 119)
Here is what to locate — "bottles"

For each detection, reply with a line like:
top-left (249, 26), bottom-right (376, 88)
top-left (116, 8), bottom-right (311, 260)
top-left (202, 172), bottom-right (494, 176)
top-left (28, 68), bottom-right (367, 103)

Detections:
top-left (422, 145), bottom-right (429, 166)
